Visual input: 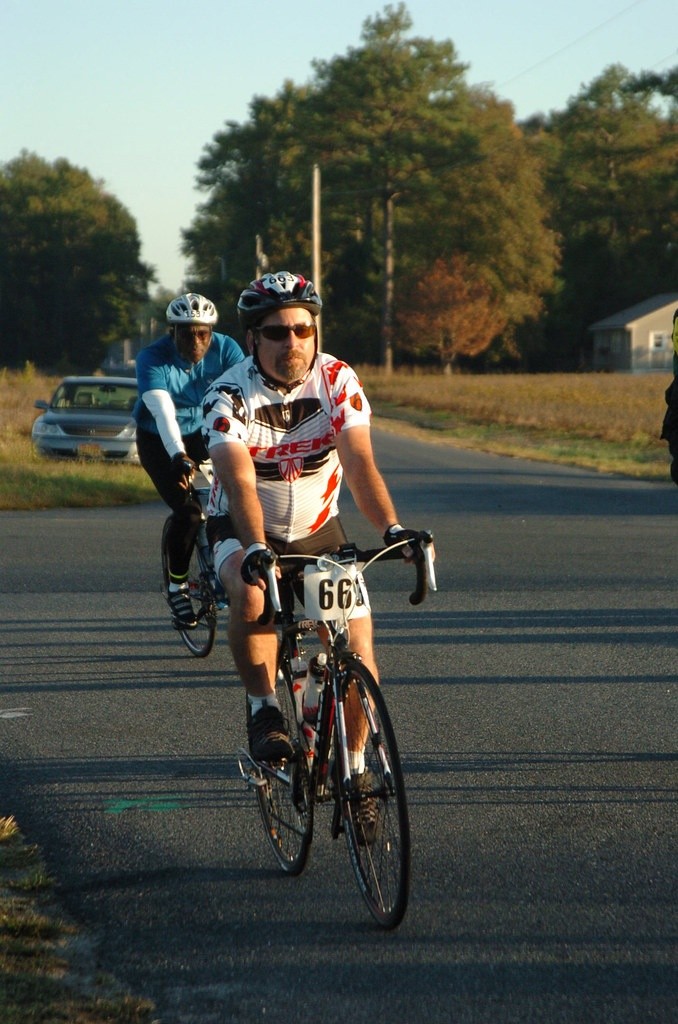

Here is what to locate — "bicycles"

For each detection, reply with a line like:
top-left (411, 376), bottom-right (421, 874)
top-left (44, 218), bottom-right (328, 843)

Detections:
top-left (159, 460), bottom-right (232, 658)
top-left (234, 529), bottom-right (438, 930)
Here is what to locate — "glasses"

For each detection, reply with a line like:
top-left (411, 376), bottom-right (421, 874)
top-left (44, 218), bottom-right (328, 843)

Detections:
top-left (174, 329), bottom-right (211, 340)
top-left (256, 323), bottom-right (317, 341)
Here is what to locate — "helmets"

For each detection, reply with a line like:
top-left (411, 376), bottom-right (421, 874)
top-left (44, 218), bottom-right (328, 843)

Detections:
top-left (237, 270), bottom-right (323, 325)
top-left (166, 293), bottom-right (218, 325)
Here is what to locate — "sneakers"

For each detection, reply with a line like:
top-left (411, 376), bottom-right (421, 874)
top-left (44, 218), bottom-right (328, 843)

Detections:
top-left (167, 581), bottom-right (198, 629)
top-left (331, 758), bottom-right (379, 845)
top-left (245, 691), bottom-right (294, 761)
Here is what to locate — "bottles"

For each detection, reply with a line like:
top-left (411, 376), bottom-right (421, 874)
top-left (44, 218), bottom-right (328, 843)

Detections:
top-left (302, 653), bottom-right (328, 739)
top-left (291, 657), bottom-right (309, 724)
top-left (198, 512), bottom-right (215, 569)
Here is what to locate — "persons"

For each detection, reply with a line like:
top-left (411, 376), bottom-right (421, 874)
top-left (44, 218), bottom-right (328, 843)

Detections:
top-left (131, 292), bottom-right (248, 624)
top-left (197, 271), bottom-right (437, 849)
top-left (659, 310), bottom-right (678, 486)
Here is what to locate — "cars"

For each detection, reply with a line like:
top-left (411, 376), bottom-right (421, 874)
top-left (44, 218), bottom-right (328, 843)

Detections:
top-left (31, 375), bottom-right (142, 468)
